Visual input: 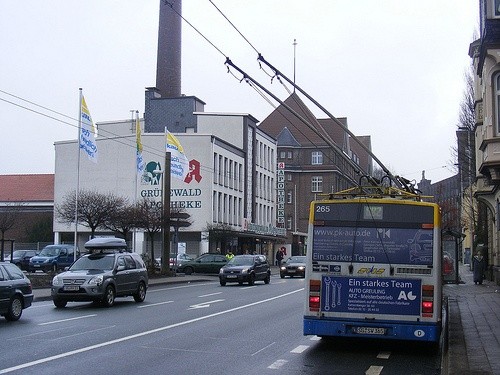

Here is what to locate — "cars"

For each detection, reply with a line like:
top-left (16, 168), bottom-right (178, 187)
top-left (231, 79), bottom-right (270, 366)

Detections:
top-left (280, 255), bottom-right (306, 279)
top-left (4, 250), bottom-right (40, 273)
top-left (177, 252), bottom-right (238, 275)
top-left (0, 262), bottom-right (34, 321)
top-left (156, 252), bottom-right (193, 270)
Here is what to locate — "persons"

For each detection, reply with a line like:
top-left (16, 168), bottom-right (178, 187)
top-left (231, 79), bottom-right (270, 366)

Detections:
top-left (226, 250), bottom-right (236, 261)
top-left (472, 251), bottom-right (485, 285)
top-left (276, 248), bottom-right (284, 269)
top-left (216, 248), bottom-right (220, 255)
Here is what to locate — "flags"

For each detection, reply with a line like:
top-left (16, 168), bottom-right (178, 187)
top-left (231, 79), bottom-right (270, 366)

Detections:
top-left (77, 90), bottom-right (98, 164)
top-left (163, 127), bottom-right (190, 177)
top-left (135, 113), bottom-right (146, 171)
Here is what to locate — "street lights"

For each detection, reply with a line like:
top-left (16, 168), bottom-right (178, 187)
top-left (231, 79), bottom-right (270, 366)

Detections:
top-left (454, 163), bottom-right (464, 265)
top-left (458, 126), bottom-right (473, 272)
top-left (153, 170), bottom-right (164, 271)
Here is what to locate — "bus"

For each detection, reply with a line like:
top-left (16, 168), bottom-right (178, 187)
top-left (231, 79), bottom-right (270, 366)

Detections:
top-left (223, 53), bottom-right (444, 343)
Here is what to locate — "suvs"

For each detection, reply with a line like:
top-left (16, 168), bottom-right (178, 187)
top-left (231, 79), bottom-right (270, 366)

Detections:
top-left (30, 244), bottom-right (80, 273)
top-left (52, 237), bottom-right (149, 308)
top-left (219, 255), bottom-right (271, 287)
